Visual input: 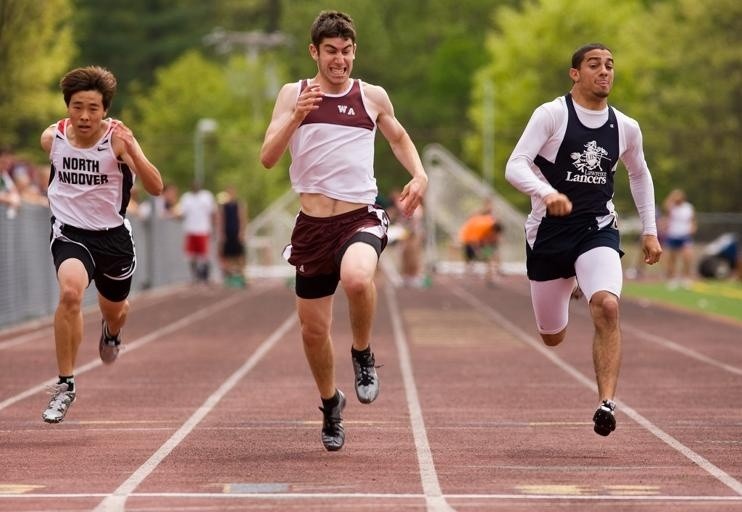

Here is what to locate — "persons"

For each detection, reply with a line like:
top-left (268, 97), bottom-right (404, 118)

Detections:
top-left (260, 10), bottom-right (429, 452)
top-left (375, 187), bottom-right (505, 288)
top-left (661, 190), bottom-right (698, 292)
top-left (502, 43), bottom-right (663, 436)
top-left (42, 65), bottom-right (165, 424)
top-left (0, 147), bottom-right (247, 291)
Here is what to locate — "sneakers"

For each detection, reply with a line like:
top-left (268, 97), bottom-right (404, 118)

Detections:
top-left (351, 344), bottom-right (379, 403)
top-left (321, 388), bottom-right (347, 452)
top-left (593, 399), bottom-right (617, 436)
top-left (99, 314), bottom-right (123, 365)
top-left (40, 381), bottom-right (77, 422)
